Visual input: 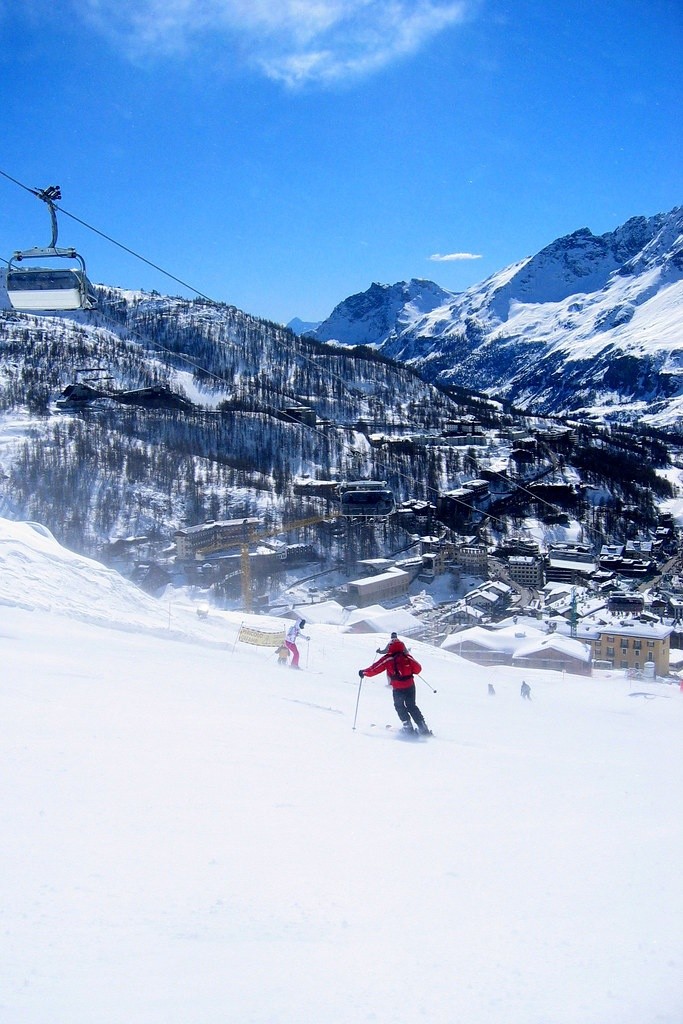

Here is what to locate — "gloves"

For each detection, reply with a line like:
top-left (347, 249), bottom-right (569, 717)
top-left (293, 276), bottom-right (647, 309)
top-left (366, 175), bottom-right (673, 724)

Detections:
top-left (376, 648), bottom-right (380, 653)
top-left (359, 670), bottom-right (364, 678)
top-left (306, 636), bottom-right (310, 640)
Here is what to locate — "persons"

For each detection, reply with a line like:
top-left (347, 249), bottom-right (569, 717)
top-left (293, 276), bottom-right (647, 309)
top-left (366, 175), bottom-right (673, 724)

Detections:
top-left (275, 645), bottom-right (290, 664)
top-left (359, 633), bottom-right (429, 737)
top-left (488, 684), bottom-right (495, 695)
top-left (521, 680), bottom-right (532, 700)
top-left (285, 619), bottom-right (310, 669)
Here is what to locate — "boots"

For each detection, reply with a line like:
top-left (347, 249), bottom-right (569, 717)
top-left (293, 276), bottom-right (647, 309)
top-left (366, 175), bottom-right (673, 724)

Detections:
top-left (399, 720), bottom-right (414, 734)
top-left (417, 720), bottom-right (429, 735)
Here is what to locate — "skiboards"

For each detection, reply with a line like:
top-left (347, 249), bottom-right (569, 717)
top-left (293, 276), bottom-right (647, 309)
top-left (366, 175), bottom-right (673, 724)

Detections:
top-left (369, 724), bottom-right (434, 743)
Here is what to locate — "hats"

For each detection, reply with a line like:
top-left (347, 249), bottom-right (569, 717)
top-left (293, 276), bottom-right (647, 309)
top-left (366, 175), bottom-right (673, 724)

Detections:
top-left (391, 632), bottom-right (397, 639)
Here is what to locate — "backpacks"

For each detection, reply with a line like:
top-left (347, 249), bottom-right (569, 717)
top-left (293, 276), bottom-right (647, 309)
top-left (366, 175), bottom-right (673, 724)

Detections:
top-left (389, 651), bottom-right (412, 681)
